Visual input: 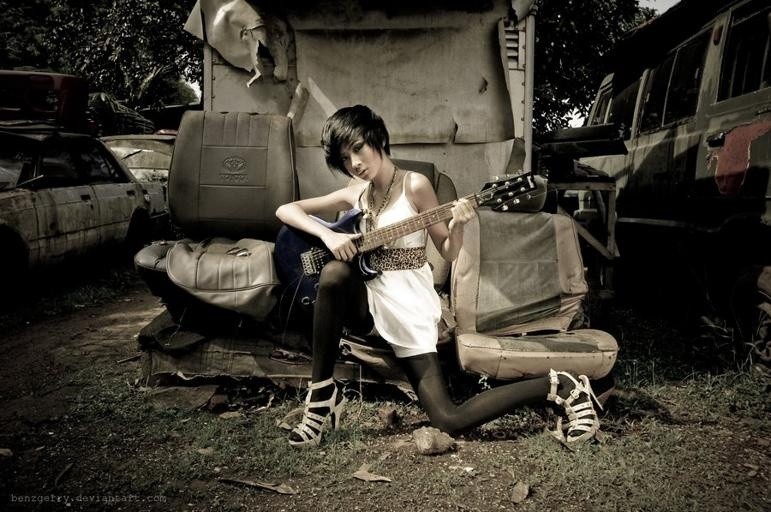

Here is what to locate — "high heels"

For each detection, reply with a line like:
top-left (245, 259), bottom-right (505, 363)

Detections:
top-left (288, 379), bottom-right (347, 451)
top-left (547, 368), bottom-right (605, 445)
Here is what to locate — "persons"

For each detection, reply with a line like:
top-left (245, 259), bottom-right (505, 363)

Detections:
top-left (274, 104), bottom-right (605, 450)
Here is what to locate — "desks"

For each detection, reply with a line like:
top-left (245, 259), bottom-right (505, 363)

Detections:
top-left (550, 176), bottom-right (616, 292)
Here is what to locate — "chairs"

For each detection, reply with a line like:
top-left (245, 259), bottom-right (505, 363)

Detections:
top-left (126, 110), bottom-right (296, 340)
top-left (334, 159), bottom-right (459, 353)
top-left (450, 173), bottom-right (620, 384)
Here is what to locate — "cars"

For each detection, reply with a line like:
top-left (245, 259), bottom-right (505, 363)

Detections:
top-left (0, 129), bottom-right (183, 297)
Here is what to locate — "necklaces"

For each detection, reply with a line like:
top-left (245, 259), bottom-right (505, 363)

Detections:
top-left (367, 163), bottom-right (397, 234)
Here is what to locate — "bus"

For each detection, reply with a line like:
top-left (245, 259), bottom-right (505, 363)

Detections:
top-left (564, 1), bottom-right (771, 228)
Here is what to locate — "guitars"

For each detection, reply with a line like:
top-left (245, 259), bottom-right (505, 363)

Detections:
top-left (274, 168), bottom-right (539, 320)
